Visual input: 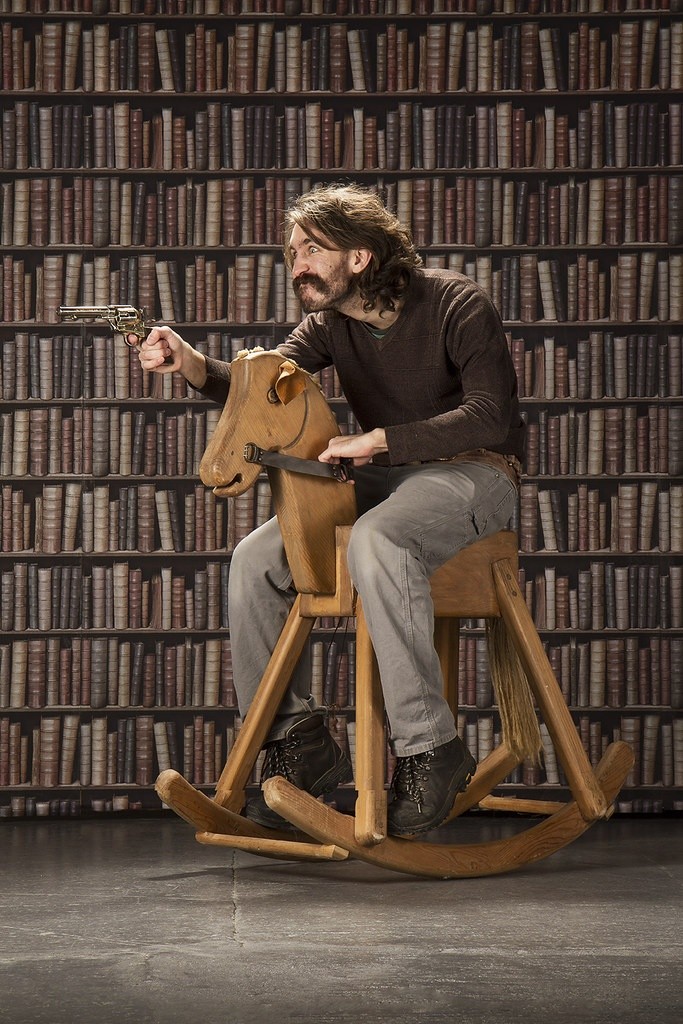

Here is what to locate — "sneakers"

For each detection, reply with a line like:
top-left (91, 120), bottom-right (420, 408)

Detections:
top-left (244, 712), bottom-right (355, 832)
top-left (385, 736), bottom-right (477, 836)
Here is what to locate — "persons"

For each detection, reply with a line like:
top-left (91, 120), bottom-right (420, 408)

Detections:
top-left (128, 184), bottom-right (525, 838)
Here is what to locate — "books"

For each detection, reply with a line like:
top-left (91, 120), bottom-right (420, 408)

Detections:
top-left (0, 0), bottom-right (683, 817)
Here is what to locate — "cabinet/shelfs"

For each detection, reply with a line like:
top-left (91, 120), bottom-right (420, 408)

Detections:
top-left (0, 9), bottom-right (683, 821)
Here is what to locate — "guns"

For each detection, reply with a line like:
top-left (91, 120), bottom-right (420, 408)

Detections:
top-left (56, 304), bottom-right (175, 367)
